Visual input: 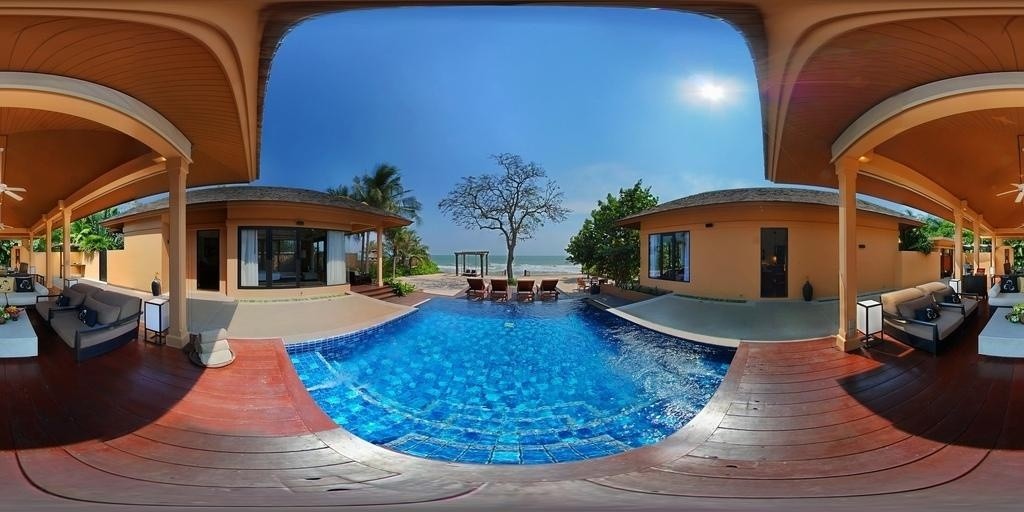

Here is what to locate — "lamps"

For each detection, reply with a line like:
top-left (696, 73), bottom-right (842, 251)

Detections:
top-left (145, 298), bottom-right (170, 345)
top-left (857, 300), bottom-right (884, 348)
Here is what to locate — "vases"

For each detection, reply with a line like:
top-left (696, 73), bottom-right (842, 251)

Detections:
top-left (152, 279), bottom-right (161, 296)
top-left (802, 281), bottom-right (813, 302)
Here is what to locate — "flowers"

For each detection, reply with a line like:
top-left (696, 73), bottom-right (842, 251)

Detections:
top-left (152, 272), bottom-right (160, 279)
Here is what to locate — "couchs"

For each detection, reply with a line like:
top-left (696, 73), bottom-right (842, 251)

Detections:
top-left (879, 275), bottom-right (1024, 358)
top-left (0, 273), bottom-right (145, 366)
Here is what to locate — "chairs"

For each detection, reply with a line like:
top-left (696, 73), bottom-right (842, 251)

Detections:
top-left (575, 277), bottom-right (608, 294)
top-left (465, 278), bottom-right (560, 304)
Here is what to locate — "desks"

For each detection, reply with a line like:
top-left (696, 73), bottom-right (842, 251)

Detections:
top-left (60, 264), bottom-right (86, 278)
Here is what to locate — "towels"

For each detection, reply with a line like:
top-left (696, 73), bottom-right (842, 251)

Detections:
top-left (192, 327), bottom-right (233, 365)
